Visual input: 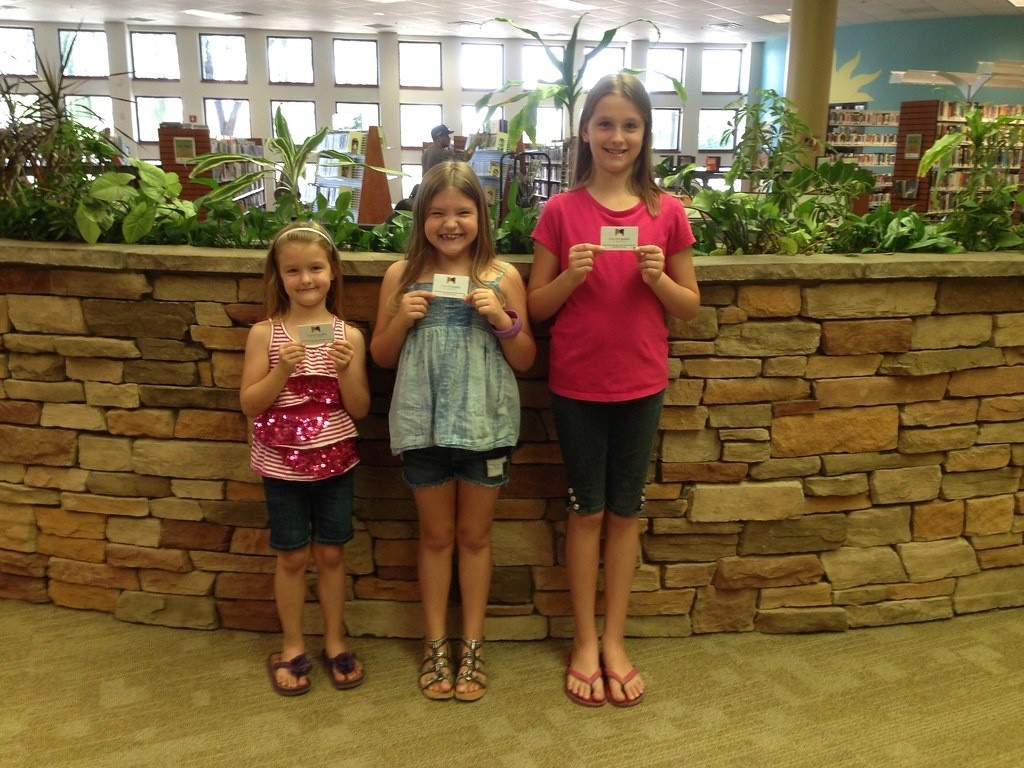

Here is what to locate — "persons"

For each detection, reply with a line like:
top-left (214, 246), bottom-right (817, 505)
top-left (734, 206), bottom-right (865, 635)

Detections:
top-left (526, 74), bottom-right (702, 706)
top-left (367, 159), bottom-right (537, 702)
top-left (238, 219), bottom-right (372, 696)
top-left (420, 125), bottom-right (483, 178)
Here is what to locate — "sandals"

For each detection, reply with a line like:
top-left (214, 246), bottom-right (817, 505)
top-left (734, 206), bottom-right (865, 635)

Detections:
top-left (419, 633), bottom-right (456, 699)
top-left (454, 632), bottom-right (488, 702)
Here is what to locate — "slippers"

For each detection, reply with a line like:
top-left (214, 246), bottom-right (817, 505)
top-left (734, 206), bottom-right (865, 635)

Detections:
top-left (267, 653), bottom-right (314, 695)
top-left (564, 651), bottom-right (607, 707)
top-left (600, 650), bottom-right (644, 706)
top-left (321, 648), bottom-right (365, 690)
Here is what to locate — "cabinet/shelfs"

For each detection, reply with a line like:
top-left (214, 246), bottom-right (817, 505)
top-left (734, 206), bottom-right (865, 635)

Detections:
top-left (159, 100), bottom-right (1024, 232)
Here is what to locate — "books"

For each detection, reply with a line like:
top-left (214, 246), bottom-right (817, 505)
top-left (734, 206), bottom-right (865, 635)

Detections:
top-left (478, 132), bottom-right (510, 205)
top-left (927, 193), bottom-right (970, 213)
top-left (935, 124), bottom-right (1024, 147)
top-left (871, 174), bottom-right (894, 185)
top-left (826, 152), bottom-right (896, 165)
top-left (932, 171), bottom-right (1019, 189)
top-left (934, 148), bottom-right (1021, 169)
top-left (210, 138), bottom-right (265, 214)
top-left (314, 132), bottom-right (362, 207)
top-left (525, 145), bottom-right (566, 217)
top-left (869, 192), bottom-right (891, 205)
top-left (827, 132), bottom-right (897, 146)
top-left (939, 100), bottom-right (1024, 122)
top-left (830, 111), bottom-right (900, 125)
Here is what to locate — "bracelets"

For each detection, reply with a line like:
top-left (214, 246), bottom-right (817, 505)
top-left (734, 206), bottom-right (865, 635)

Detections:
top-left (491, 309), bottom-right (522, 338)
